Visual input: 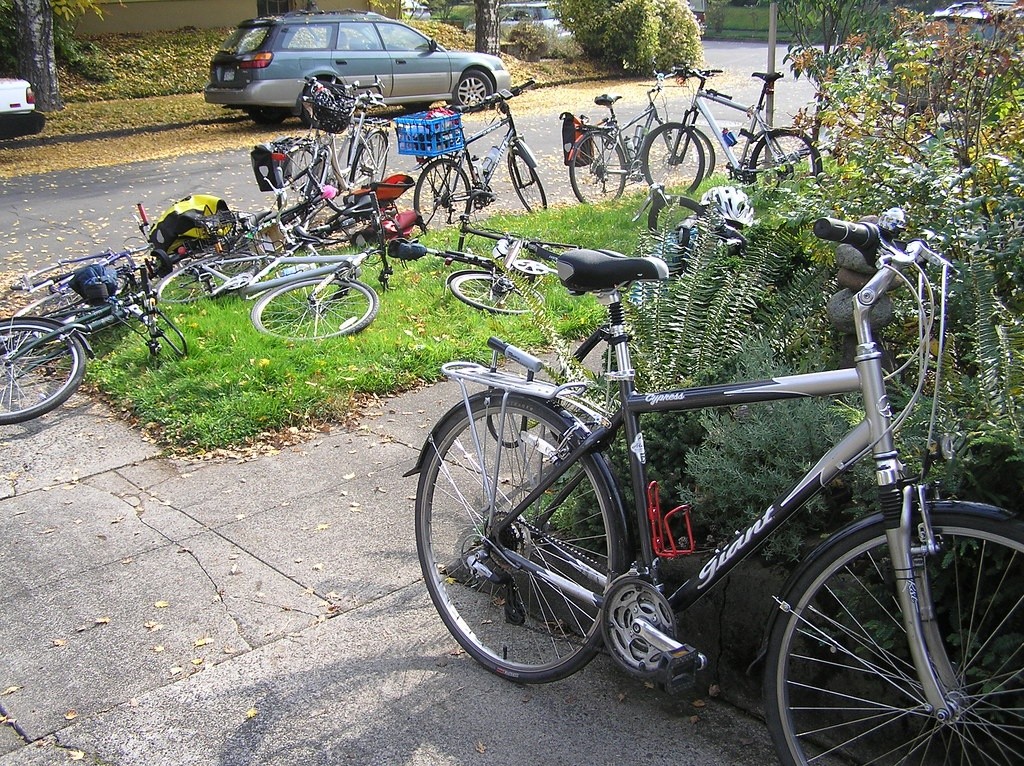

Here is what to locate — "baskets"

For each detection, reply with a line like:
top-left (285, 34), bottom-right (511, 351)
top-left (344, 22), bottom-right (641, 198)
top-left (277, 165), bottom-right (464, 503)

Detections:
top-left (299, 91), bottom-right (356, 134)
top-left (395, 112), bottom-right (464, 156)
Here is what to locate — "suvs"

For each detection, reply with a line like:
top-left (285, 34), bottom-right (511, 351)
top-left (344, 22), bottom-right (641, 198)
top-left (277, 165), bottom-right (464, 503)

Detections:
top-left (204, 8), bottom-right (513, 132)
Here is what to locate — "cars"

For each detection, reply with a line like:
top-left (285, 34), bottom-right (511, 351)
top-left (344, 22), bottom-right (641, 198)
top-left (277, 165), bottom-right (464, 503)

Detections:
top-left (0, 72), bottom-right (36, 116)
top-left (686, 0), bottom-right (708, 40)
top-left (401, 0), bottom-right (431, 21)
top-left (887, 1), bottom-right (1024, 112)
top-left (463, 1), bottom-right (583, 60)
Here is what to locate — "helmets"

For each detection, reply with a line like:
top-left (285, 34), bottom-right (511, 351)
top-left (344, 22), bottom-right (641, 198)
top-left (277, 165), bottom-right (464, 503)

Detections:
top-left (699, 186), bottom-right (754, 227)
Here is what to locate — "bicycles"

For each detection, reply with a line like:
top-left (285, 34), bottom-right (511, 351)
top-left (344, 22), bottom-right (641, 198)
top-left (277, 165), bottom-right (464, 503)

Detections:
top-left (640, 63), bottom-right (824, 208)
top-left (386, 189), bottom-right (589, 317)
top-left (399, 204), bottom-right (1024, 766)
top-left (565, 54), bottom-right (716, 207)
top-left (408, 77), bottom-right (550, 237)
top-left (0, 71), bottom-right (423, 427)
top-left (632, 181), bottom-right (816, 326)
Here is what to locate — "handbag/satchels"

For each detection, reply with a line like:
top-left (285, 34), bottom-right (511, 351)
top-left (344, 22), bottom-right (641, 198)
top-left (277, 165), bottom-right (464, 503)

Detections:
top-left (559, 112), bottom-right (594, 167)
top-left (251, 136), bottom-right (294, 191)
top-left (149, 195), bottom-right (237, 255)
